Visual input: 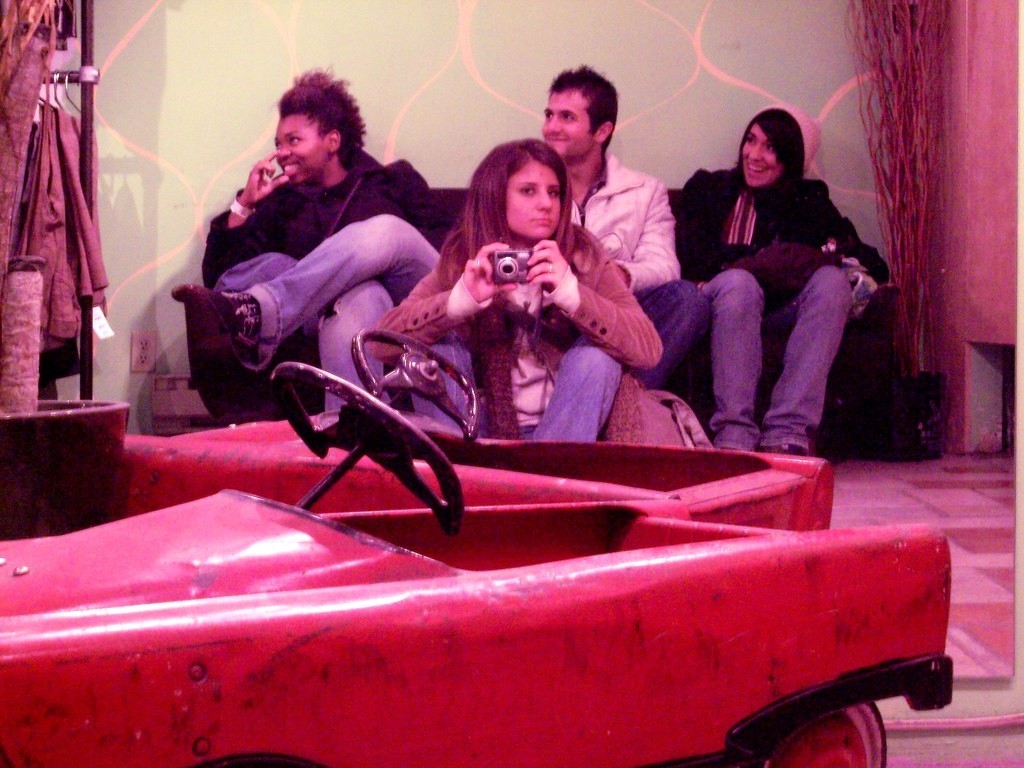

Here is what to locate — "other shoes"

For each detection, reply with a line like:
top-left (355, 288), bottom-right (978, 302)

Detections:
top-left (171, 284), bottom-right (261, 376)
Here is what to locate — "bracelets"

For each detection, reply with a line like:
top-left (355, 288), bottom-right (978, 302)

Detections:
top-left (230, 198), bottom-right (256, 217)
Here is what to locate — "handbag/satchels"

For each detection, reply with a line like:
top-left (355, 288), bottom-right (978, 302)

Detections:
top-left (858, 373), bottom-right (950, 461)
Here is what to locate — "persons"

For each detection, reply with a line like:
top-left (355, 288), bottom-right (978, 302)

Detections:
top-left (371, 138), bottom-right (663, 442)
top-left (540, 65), bottom-right (710, 389)
top-left (172, 72), bottom-right (443, 412)
top-left (673, 105), bottom-right (891, 457)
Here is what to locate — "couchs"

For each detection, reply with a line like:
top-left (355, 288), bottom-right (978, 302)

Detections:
top-left (185, 188), bottom-right (898, 456)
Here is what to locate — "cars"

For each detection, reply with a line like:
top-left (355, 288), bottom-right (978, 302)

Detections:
top-left (1, 320), bottom-right (955, 768)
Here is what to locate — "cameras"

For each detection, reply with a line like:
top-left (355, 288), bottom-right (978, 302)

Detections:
top-left (493, 248), bottom-right (542, 284)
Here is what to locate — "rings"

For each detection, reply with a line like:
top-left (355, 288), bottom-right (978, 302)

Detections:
top-left (549, 264), bottom-right (552, 271)
top-left (477, 260), bottom-right (479, 265)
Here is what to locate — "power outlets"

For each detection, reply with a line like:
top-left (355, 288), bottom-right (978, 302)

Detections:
top-left (131, 330), bottom-right (157, 373)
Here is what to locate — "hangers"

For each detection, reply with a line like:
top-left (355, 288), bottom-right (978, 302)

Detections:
top-left (31, 68), bottom-right (83, 121)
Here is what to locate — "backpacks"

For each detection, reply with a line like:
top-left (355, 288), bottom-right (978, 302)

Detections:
top-left (602, 375), bottom-right (714, 449)
